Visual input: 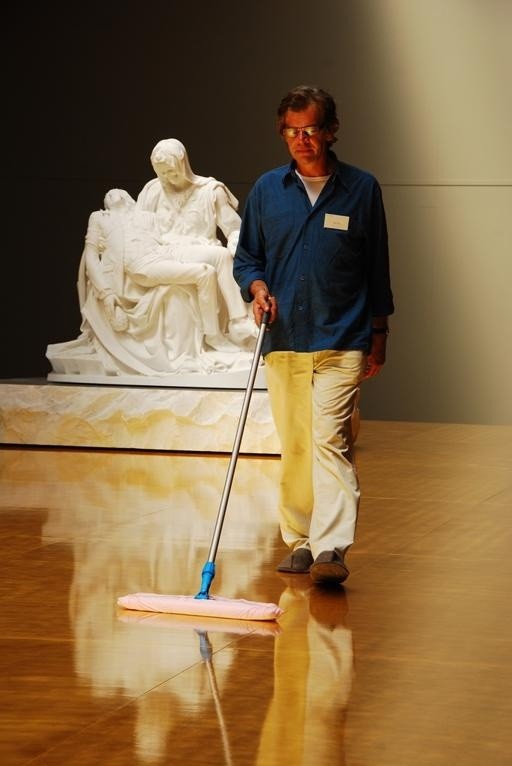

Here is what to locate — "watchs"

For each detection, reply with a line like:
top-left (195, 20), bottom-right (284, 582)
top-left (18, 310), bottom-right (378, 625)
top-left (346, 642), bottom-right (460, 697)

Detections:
top-left (370, 325), bottom-right (389, 337)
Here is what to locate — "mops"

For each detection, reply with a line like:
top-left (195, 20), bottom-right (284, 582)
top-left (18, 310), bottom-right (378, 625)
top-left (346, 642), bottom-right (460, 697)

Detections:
top-left (116, 300), bottom-right (285, 622)
top-left (111, 612), bottom-right (279, 764)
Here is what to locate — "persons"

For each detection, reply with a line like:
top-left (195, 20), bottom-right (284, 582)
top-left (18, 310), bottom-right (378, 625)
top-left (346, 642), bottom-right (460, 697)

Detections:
top-left (132, 136), bottom-right (245, 371)
top-left (82, 186), bottom-right (263, 357)
top-left (251, 566), bottom-right (354, 765)
top-left (130, 503), bottom-right (245, 764)
top-left (69, 541), bottom-right (261, 766)
top-left (233, 84), bottom-right (396, 590)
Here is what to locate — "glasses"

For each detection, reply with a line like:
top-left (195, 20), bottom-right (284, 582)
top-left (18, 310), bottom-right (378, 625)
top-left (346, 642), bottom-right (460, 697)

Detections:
top-left (281, 120), bottom-right (327, 138)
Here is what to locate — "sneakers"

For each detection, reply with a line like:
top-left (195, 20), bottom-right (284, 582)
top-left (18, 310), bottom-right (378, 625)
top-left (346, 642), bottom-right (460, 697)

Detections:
top-left (276, 548), bottom-right (314, 573)
top-left (309, 551), bottom-right (349, 583)
